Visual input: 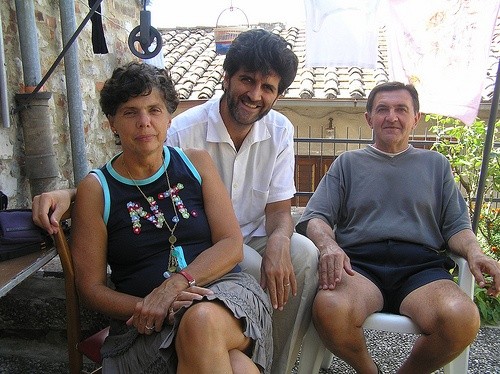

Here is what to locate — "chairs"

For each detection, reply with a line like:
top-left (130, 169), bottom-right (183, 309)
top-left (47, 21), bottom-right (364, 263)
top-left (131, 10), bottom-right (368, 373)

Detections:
top-left (48, 201), bottom-right (110, 374)
top-left (296, 251), bottom-right (475, 374)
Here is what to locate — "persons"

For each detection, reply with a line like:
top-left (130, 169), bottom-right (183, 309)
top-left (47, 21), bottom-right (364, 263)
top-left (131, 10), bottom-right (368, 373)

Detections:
top-left (295, 82), bottom-right (500, 374)
top-left (72, 61), bottom-right (273, 374)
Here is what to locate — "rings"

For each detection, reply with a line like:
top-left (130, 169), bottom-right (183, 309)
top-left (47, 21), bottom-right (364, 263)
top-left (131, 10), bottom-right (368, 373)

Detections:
top-left (284, 283), bottom-right (290, 287)
top-left (145, 324), bottom-right (156, 330)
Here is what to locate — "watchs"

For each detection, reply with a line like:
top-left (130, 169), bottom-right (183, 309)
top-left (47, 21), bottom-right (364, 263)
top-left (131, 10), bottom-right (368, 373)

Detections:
top-left (179, 270), bottom-right (196, 287)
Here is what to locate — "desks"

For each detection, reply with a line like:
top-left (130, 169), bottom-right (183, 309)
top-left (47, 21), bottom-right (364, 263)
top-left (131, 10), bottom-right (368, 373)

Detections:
top-left (1, 233), bottom-right (69, 303)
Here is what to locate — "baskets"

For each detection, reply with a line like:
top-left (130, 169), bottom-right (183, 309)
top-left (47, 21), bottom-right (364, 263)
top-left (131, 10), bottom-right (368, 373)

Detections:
top-left (212, 7), bottom-right (250, 55)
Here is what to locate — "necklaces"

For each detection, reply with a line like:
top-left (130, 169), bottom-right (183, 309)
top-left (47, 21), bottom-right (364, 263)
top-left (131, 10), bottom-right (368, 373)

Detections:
top-left (120, 153), bottom-right (178, 273)
top-left (32, 29), bottom-right (322, 374)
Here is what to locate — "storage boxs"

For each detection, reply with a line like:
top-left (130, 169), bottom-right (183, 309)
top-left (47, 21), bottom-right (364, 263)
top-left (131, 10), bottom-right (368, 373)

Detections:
top-left (214, 27), bottom-right (242, 55)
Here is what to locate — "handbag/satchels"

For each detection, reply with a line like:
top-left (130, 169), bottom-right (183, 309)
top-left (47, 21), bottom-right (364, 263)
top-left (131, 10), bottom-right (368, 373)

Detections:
top-left (0, 209), bottom-right (56, 260)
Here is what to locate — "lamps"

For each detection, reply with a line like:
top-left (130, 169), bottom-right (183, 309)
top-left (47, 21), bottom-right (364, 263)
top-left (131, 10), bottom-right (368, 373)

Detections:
top-left (324, 116), bottom-right (336, 139)
